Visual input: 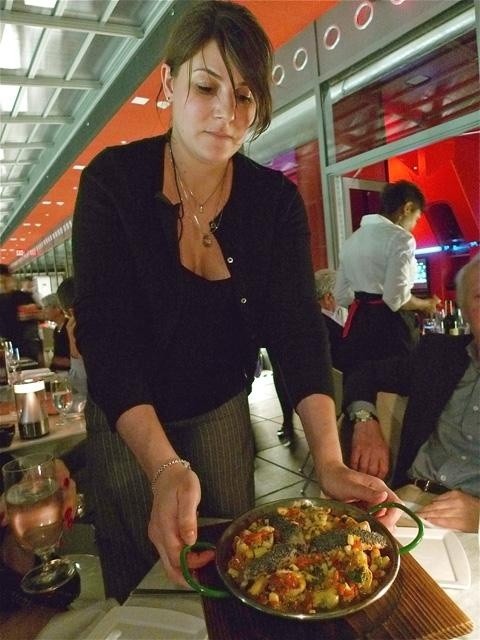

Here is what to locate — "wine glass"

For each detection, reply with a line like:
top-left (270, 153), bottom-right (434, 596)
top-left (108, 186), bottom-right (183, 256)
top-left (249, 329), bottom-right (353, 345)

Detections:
top-left (50, 377), bottom-right (88, 426)
top-left (1, 340), bottom-right (22, 384)
top-left (1, 453), bottom-right (80, 596)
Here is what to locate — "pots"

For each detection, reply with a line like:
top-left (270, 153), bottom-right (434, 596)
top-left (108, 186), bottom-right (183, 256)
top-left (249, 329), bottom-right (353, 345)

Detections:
top-left (178, 494), bottom-right (425, 623)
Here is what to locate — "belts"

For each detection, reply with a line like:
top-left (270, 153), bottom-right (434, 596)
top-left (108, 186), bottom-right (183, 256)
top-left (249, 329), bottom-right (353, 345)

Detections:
top-left (405, 477), bottom-right (450, 495)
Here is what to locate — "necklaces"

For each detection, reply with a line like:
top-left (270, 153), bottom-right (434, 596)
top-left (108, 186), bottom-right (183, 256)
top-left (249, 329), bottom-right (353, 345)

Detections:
top-left (168, 149), bottom-right (227, 247)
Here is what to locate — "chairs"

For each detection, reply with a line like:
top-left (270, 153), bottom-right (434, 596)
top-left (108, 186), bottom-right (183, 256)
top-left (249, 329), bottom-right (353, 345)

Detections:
top-left (299, 368), bottom-right (344, 497)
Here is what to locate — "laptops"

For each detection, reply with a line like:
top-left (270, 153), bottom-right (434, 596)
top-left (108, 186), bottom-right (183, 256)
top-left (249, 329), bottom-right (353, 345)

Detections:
top-left (410, 256), bottom-right (432, 299)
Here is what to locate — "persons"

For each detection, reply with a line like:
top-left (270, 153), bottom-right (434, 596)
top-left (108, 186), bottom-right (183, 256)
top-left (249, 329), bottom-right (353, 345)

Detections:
top-left (71, 1), bottom-right (404, 605)
top-left (343, 252), bottom-right (479, 531)
top-left (331, 179), bottom-right (441, 373)
top-left (0, 263), bottom-right (89, 394)
top-left (314, 268), bottom-right (349, 346)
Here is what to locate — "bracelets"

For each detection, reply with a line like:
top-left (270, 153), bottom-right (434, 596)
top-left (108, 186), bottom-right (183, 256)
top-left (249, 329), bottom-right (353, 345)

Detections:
top-left (150, 459), bottom-right (191, 493)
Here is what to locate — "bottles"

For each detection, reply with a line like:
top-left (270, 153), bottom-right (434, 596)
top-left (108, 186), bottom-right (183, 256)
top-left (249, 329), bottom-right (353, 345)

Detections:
top-left (441, 299), bottom-right (459, 336)
top-left (13, 379), bottom-right (50, 440)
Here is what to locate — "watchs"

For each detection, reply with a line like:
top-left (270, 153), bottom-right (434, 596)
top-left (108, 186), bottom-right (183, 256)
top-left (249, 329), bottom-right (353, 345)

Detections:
top-left (352, 409), bottom-right (380, 424)
